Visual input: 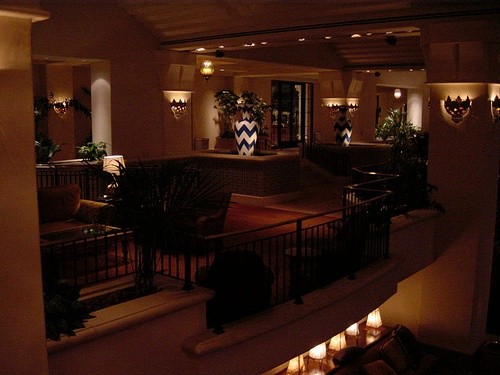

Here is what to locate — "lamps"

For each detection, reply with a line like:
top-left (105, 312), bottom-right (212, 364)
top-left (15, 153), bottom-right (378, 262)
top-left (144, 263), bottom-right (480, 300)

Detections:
top-left (287, 308), bottom-right (382, 375)
top-left (444, 96), bottom-right (472, 123)
top-left (171, 100), bottom-right (188, 117)
top-left (102, 155), bottom-right (126, 196)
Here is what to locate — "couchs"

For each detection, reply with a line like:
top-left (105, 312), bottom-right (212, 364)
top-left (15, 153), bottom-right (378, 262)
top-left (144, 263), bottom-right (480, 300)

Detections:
top-left (38, 184), bottom-right (115, 256)
top-left (167, 164), bottom-right (234, 253)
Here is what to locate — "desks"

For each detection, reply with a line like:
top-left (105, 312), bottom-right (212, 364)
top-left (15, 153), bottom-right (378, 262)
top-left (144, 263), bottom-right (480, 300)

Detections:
top-left (39, 222), bottom-right (133, 271)
top-left (283, 248), bottom-right (322, 300)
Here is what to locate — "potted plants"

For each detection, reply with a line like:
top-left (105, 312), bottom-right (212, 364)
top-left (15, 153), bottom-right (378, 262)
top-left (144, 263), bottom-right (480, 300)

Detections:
top-left (214, 88), bottom-right (271, 151)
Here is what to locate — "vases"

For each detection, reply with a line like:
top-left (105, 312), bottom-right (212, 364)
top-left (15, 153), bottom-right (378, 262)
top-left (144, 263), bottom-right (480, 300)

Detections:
top-left (332, 106), bottom-right (353, 144)
top-left (234, 104), bottom-right (259, 157)
